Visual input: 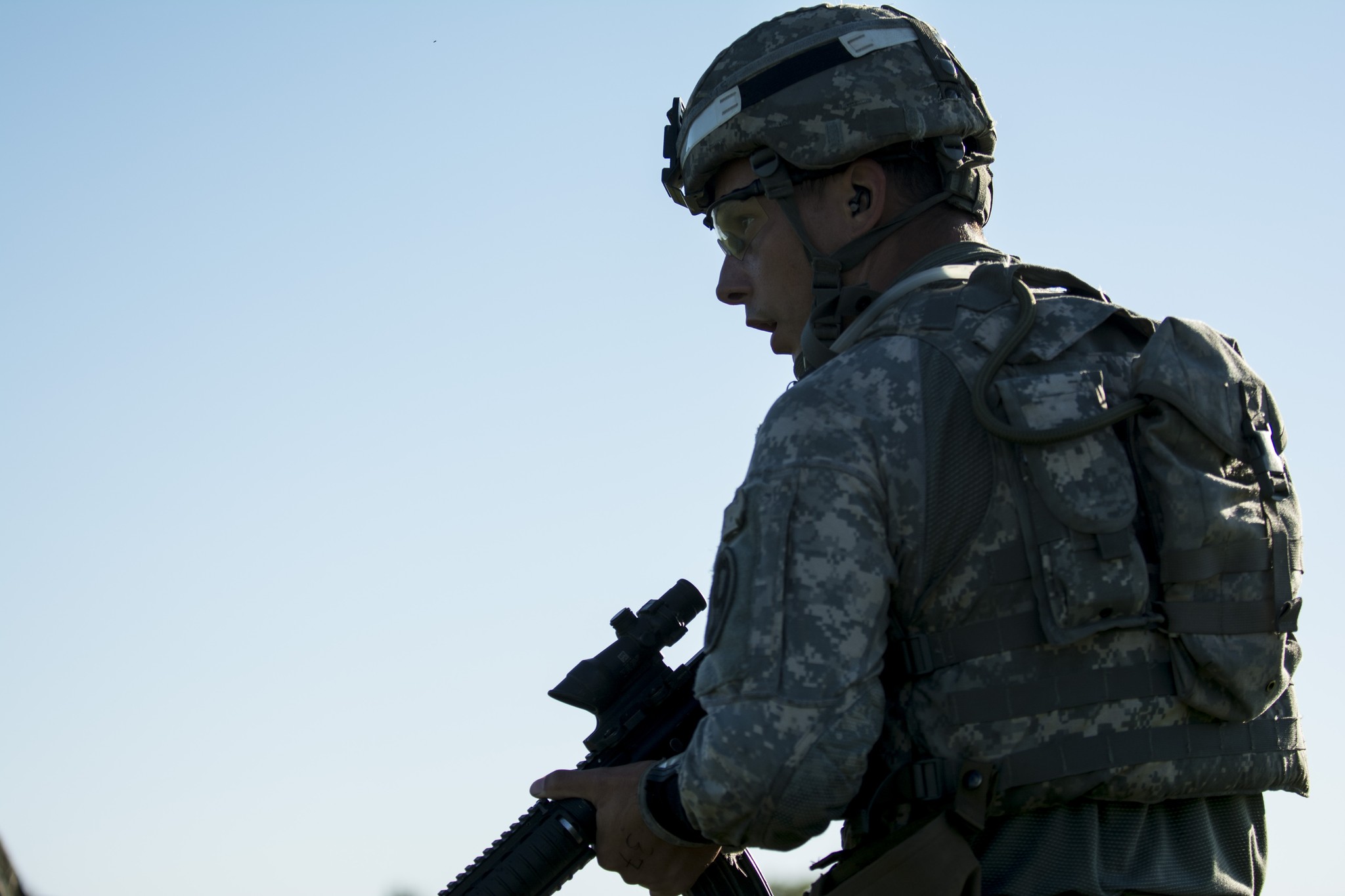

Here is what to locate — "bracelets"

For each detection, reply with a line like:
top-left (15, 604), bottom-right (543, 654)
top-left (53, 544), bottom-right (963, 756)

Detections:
top-left (637, 753), bottom-right (720, 849)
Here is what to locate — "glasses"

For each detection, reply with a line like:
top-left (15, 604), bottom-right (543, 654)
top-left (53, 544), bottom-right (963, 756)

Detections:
top-left (712, 196), bottom-right (768, 261)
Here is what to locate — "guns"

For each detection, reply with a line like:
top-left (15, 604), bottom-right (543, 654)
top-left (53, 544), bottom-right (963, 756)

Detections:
top-left (438, 577), bottom-right (780, 896)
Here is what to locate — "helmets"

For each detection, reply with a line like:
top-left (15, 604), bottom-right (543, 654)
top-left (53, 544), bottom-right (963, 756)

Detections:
top-left (676, 2), bottom-right (998, 216)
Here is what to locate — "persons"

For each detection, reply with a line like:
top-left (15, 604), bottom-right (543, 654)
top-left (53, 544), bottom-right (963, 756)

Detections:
top-left (529, 3), bottom-right (1309, 894)
